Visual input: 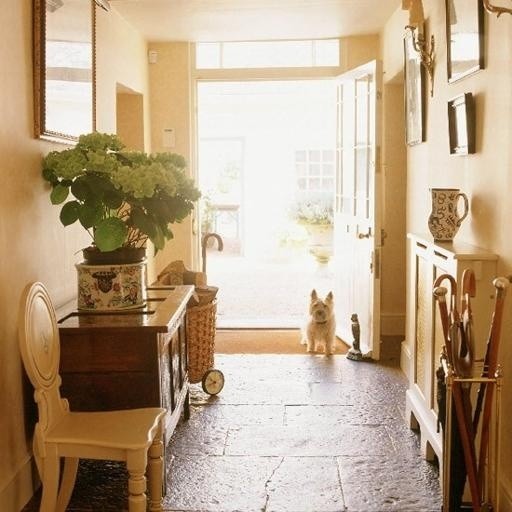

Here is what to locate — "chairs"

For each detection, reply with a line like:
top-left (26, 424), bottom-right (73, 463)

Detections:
top-left (18, 281), bottom-right (170, 511)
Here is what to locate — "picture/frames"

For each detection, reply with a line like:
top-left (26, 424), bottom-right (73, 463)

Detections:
top-left (444, 0), bottom-right (487, 86)
top-left (32, 1), bottom-right (100, 148)
top-left (402, 22), bottom-right (426, 147)
top-left (447, 92), bottom-right (475, 156)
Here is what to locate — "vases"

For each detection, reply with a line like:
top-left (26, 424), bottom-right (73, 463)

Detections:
top-left (428, 188), bottom-right (470, 242)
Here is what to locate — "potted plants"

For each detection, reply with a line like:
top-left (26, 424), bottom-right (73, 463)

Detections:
top-left (37, 130), bottom-right (200, 311)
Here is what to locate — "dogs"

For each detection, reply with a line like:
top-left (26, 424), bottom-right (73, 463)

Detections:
top-left (301, 289), bottom-right (338, 357)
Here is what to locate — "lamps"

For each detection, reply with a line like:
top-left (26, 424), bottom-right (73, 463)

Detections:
top-left (400, 0), bottom-right (436, 97)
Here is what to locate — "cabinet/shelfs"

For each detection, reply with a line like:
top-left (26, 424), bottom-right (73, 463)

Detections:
top-left (53, 285), bottom-right (196, 496)
top-left (400, 231), bottom-right (500, 508)
